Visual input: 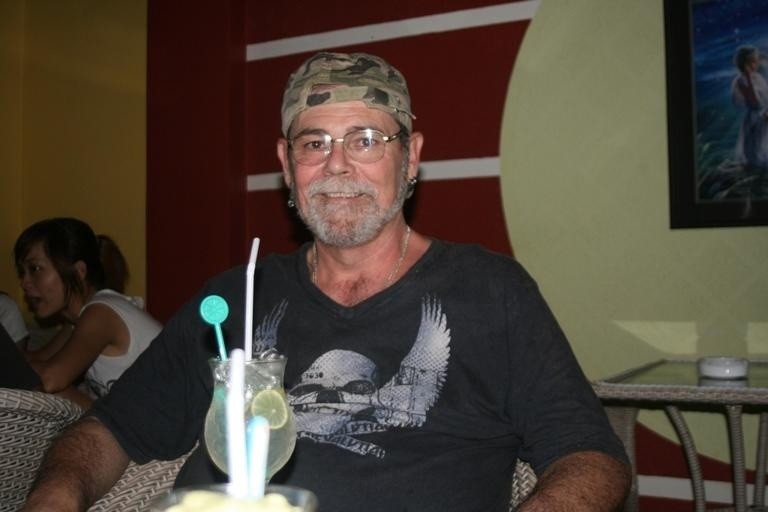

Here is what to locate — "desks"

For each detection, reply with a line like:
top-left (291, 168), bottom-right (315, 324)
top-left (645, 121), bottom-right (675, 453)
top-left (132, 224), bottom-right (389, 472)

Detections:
top-left (591, 354), bottom-right (768, 511)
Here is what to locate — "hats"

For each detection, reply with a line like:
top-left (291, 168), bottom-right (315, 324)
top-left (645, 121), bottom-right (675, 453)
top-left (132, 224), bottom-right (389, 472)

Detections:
top-left (281, 52), bottom-right (417, 138)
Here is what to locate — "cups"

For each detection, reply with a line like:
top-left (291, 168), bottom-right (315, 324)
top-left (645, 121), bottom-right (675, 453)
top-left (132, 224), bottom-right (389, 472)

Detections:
top-left (147, 484), bottom-right (319, 512)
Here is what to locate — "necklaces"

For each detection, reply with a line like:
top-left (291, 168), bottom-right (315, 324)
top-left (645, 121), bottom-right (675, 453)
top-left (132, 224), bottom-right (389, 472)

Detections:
top-left (310, 224), bottom-right (413, 287)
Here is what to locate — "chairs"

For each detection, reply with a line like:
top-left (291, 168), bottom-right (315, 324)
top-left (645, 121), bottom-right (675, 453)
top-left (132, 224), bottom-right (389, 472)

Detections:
top-left (508, 457), bottom-right (537, 510)
top-left (0, 386), bottom-right (84, 511)
top-left (88, 439), bottom-right (205, 510)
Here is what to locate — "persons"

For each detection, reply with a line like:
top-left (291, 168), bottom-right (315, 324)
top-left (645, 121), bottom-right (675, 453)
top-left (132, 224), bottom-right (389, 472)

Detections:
top-left (730, 47), bottom-right (768, 168)
top-left (14, 218), bottom-right (164, 399)
top-left (0, 324), bottom-right (43, 391)
top-left (1, 293), bottom-right (29, 348)
top-left (18, 51), bottom-right (628, 511)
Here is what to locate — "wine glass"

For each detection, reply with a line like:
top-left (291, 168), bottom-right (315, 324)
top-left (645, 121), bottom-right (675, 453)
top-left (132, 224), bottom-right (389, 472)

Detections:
top-left (203, 352), bottom-right (297, 483)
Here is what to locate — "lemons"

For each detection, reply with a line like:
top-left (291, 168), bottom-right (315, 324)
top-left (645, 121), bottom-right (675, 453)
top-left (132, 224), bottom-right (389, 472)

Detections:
top-left (251, 388), bottom-right (288, 430)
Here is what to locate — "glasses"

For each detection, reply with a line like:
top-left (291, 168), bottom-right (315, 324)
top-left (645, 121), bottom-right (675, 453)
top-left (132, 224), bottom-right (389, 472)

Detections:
top-left (287, 129), bottom-right (405, 167)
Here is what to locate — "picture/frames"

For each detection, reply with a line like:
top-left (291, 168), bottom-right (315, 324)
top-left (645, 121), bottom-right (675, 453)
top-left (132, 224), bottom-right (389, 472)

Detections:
top-left (660, 1), bottom-right (766, 233)
